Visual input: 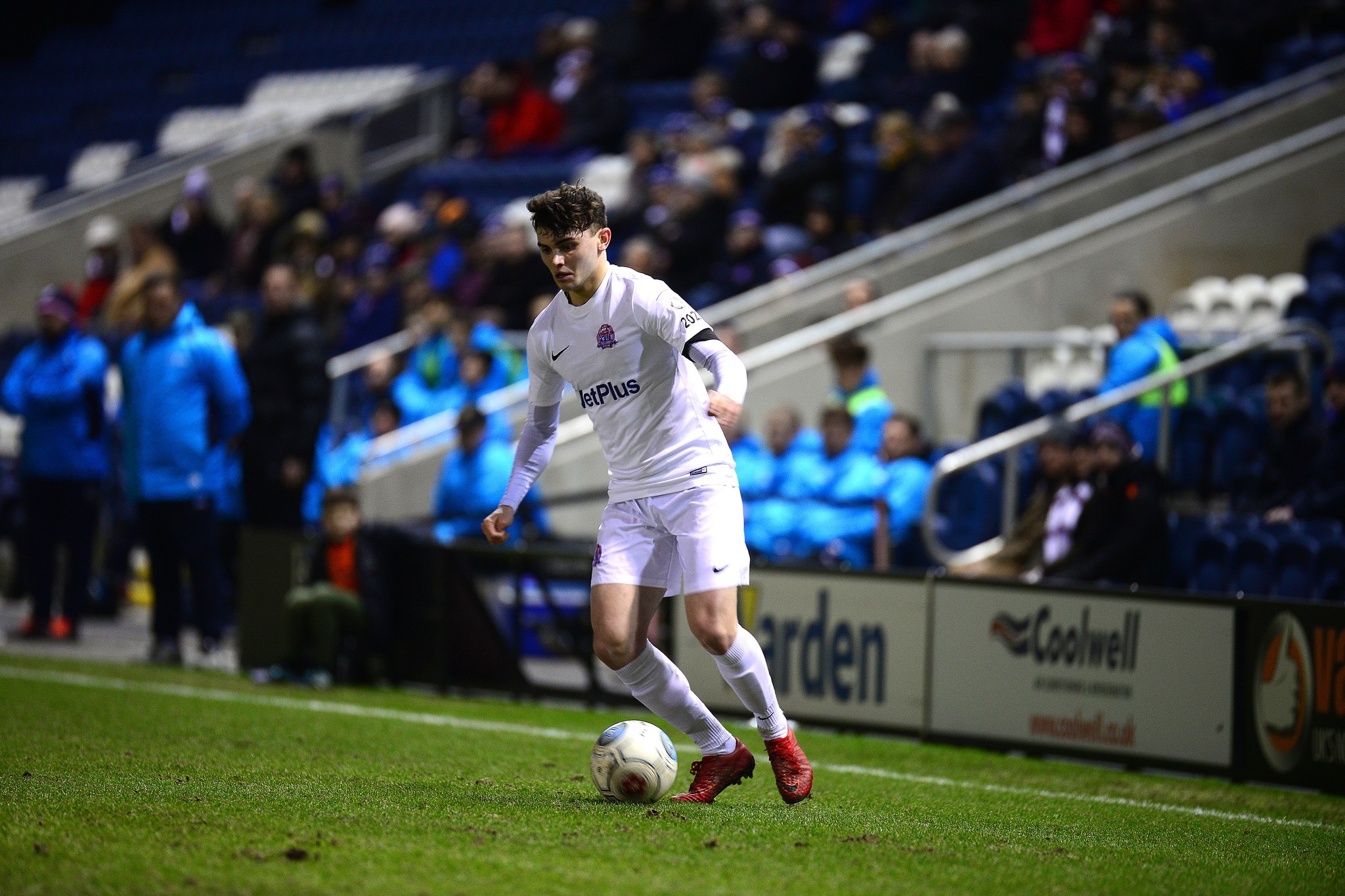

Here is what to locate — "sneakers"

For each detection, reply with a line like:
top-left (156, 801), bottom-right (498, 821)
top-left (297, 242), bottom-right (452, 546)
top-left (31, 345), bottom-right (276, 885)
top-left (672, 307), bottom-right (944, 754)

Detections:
top-left (766, 725), bottom-right (814, 803)
top-left (669, 738), bottom-right (754, 803)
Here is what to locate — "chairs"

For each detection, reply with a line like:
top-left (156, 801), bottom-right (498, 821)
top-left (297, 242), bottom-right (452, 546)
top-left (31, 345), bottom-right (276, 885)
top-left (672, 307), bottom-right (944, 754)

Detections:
top-left (1, 5), bottom-right (1345, 637)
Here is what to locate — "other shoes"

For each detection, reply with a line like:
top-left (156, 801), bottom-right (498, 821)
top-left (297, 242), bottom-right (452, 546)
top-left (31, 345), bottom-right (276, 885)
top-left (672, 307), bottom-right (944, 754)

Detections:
top-left (41, 620), bottom-right (77, 641)
top-left (128, 640), bottom-right (183, 663)
top-left (6, 616), bottom-right (42, 639)
top-left (187, 644), bottom-right (236, 669)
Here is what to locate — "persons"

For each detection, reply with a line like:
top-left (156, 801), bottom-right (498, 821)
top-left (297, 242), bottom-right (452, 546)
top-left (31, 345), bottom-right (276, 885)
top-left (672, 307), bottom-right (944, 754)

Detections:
top-left (475, 181), bottom-right (816, 808)
top-left (5, 0), bottom-right (1341, 509)
top-left (431, 407), bottom-right (552, 591)
top-left (701, 236), bottom-right (1345, 607)
top-left (255, 488), bottom-right (395, 693)
top-left (1, 280), bottom-right (124, 650)
top-left (111, 273), bottom-right (260, 678)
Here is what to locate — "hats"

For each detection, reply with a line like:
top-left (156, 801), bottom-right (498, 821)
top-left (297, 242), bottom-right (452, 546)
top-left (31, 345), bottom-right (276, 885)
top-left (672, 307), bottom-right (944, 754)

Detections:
top-left (36, 285), bottom-right (76, 307)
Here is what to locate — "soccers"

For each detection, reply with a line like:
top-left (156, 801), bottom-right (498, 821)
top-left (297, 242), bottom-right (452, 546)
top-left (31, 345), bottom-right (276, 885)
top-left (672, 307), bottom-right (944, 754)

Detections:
top-left (589, 719), bottom-right (681, 804)
